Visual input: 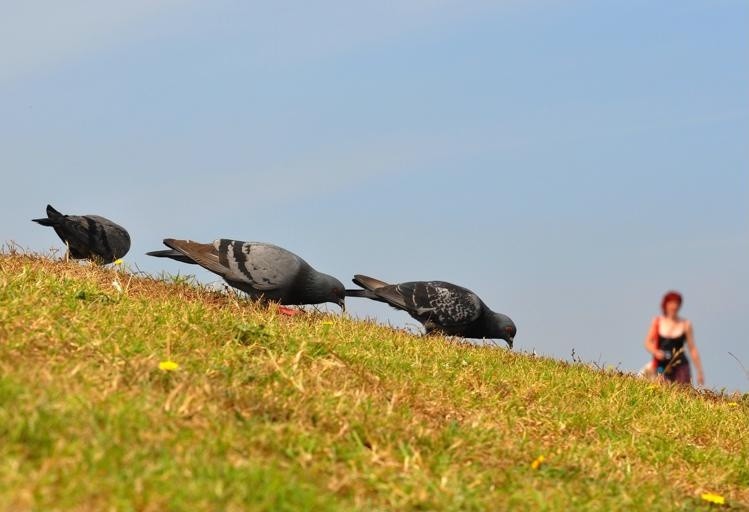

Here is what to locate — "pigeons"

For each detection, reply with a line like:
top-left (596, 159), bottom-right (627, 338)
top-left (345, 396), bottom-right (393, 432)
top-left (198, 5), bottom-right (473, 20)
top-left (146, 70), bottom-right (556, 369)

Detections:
top-left (143, 236), bottom-right (346, 316)
top-left (344, 273), bottom-right (517, 350)
top-left (30, 204), bottom-right (131, 269)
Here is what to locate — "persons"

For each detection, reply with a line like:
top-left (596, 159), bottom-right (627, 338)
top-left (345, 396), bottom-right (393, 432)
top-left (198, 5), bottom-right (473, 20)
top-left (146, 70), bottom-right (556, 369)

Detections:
top-left (642, 291), bottom-right (705, 386)
